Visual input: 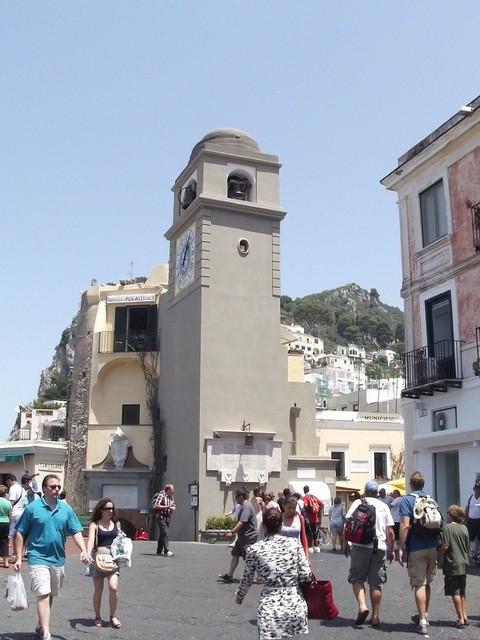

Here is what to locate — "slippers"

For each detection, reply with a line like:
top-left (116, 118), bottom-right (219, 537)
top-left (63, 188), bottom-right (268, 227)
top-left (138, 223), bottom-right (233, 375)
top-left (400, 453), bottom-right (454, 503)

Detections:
top-left (355, 610), bottom-right (369, 625)
top-left (3, 565), bottom-right (9, 568)
top-left (370, 617), bottom-right (380, 627)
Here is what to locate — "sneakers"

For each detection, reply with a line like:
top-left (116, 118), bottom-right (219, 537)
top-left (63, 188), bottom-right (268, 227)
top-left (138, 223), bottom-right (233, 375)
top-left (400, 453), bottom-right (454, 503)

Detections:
top-left (11, 553), bottom-right (16, 557)
top-left (216, 574), bottom-right (233, 583)
top-left (42, 633), bottom-right (51, 640)
top-left (308, 548), bottom-right (313, 553)
top-left (411, 615), bottom-right (429, 626)
top-left (411, 624), bottom-right (429, 635)
top-left (156, 552), bottom-right (164, 556)
top-left (252, 577), bottom-right (263, 584)
top-left (228, 542), bottom-right (236, 548)
top-left (314, 547), bottom-right (320, 553)
top-left (9, 558), bottom-right (17, 563)
top-left (36, 625), bottom-right (43, 636)
top-left (332, 549), bottom-right (336, 551)
top-left (164, 550), bottom-right (175, 557)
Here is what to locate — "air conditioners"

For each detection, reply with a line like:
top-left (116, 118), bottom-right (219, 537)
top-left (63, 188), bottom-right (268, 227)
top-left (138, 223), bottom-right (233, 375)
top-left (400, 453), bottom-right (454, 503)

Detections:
top-left (433, 408), bottom-right (456, 432)
top-left (411, 356), bottom-right (436, 384)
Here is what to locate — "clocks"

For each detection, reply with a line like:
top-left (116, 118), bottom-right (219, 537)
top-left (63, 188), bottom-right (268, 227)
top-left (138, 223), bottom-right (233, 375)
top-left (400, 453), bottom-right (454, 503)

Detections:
top-left (174, 220), bottom-right (195, 295)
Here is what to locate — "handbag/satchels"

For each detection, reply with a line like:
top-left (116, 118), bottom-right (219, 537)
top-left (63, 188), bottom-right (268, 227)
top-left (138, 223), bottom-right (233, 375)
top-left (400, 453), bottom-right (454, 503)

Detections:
top-left (305, 517), bottom-right (313, 540)
top-left (111, 536), bottom-right (129, 561)
top-left (299, 571), bottom-right (339, 620)
top-left (465, 506), bottom-right (470, 523)
top-left (95, 552), bottom-right (119, 573)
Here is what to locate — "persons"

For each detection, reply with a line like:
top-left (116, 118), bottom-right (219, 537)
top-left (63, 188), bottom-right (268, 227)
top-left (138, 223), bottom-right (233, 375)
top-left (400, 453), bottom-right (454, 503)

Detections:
top-left (280, 496), bottom-right (311, 566)
top-left (292, 493), bottom-right (304, 516)
top-left (23, 479), bottom-right (40, 558)
top-left (398, 471), bottom-right (444, 635)
top-left (261, 487), bottom-right (284, 511)
top-left (13, 474), bottom-right (89, 640)
top-left (86, 498), bottom-right (126, 628)
top-left (341, 479), bottom-right (395, 626)
top-left (438, 505), bottom-right (471, 629)
top-left (134, 526), bottom-right (149, 541)
top-left (348, 491), bottom-right (361, 510)
top-left (284, 488), bottom-right (291, 496)
top-left (389, 489), bottom-right (402, 551)
top-left (0, 484), bottom-right (13, 568)
top-left (377, 488), bottom-right (393, 508)
top-left (251, 488), bottom-right (265, 541)
top-left (19, 474), bottom-right (32, 492)
top-left (302, 485), bottom-right (324, 553)
top-left (234, 507), bottom-right (314, 640)
top-left (5, 473), bottom-right (26, 564)
top-left (225, 486), bottom-right (250, 548)
top-left (218, 488), bottom-right (263, 584)
top-left (328, 496), bottom-right (346, 551)
top-left (153, 483), bottom-right (176, 557)
top-left (463, 481), bottom-right (480, 566)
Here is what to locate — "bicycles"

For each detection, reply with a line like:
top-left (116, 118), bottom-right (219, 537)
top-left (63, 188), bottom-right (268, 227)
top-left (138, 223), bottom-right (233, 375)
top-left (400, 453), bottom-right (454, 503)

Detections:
top-left (317, 527), bottom-right (332, 546)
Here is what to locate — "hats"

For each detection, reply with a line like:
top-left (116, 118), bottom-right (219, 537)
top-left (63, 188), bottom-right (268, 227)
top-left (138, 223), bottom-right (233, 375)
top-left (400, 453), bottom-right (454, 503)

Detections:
top-left (22, 474), bottom-right (32, 484)
top-left (29, 481), bottom-right (39, 492)
top-left (475, 483), bottom-right (480, 489)
top-left (365, 481), bottom-right (378, 492)
top-left (413, 506), bottom-right (424, 519)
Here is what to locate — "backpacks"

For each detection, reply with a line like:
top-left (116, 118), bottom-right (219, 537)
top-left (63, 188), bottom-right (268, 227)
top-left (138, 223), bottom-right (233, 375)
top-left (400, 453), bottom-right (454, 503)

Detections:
top-left (406, 492), bottom-right (444, 538)
top-left (148, 491), bottom-right (165, 514)
top-left (345, 498), bottom-right (378, 554)
top-left (306, 494), bottom-right (320, 514)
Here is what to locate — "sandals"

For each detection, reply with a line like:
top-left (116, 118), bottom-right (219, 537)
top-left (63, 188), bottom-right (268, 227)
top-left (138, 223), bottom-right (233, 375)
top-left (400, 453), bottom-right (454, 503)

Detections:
top-left (449, 622), bottom-right (464, 629)
top-left (95, 618), bottom-right (103, 627)
top-left (110, 615), bottom-right (122, 629)
top-left (457, 619), bottom-right (470, 625)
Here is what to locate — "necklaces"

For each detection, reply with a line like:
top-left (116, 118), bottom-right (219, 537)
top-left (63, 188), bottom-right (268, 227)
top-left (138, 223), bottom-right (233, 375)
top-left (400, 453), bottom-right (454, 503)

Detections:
top-left (100, 520), bottom-right (111, 530)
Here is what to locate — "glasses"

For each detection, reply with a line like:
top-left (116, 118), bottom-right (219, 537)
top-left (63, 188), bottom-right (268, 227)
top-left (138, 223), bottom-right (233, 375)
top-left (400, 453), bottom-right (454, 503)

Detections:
top-left (50, 485), bottom-right (61, 490)
top-left (104, 508), bottom-right (114, 510)
top-left (22, 479), bottom-right (29, 484)
